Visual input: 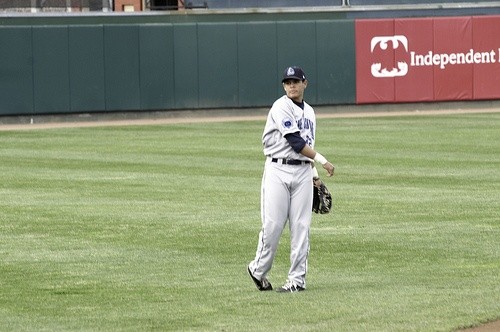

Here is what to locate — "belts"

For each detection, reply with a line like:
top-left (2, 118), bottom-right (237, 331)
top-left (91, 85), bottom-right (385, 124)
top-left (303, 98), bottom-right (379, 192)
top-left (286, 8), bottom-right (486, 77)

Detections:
top-left (271, 158), bottom-right (310, 165)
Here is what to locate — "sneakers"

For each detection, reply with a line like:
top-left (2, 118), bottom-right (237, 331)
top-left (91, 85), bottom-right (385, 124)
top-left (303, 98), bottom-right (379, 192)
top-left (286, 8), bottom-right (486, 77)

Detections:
top-left (248, 266), bottom-right (273, 291)
top-left (276, 282), bottom-right (305, 293)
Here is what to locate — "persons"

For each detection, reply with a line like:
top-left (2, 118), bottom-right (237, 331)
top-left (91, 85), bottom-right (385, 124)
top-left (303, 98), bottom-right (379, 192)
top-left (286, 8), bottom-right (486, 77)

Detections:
top-left (247, 66), bottom-right (334, 294)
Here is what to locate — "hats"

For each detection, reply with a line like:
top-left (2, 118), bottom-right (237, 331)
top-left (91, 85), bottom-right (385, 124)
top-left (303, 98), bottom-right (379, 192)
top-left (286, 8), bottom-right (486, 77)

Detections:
top-left (282, 66), bottom-right (306, 82)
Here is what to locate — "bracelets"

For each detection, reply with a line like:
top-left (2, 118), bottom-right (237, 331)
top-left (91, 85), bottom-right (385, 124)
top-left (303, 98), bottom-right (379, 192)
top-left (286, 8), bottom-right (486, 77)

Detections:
top-left (313, 152), bottom-right (327, 165)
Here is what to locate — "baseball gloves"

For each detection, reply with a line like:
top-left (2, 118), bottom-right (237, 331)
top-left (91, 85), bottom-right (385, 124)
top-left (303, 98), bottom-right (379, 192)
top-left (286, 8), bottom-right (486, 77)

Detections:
top-left (312, 179), bottom-right (332, 215)
